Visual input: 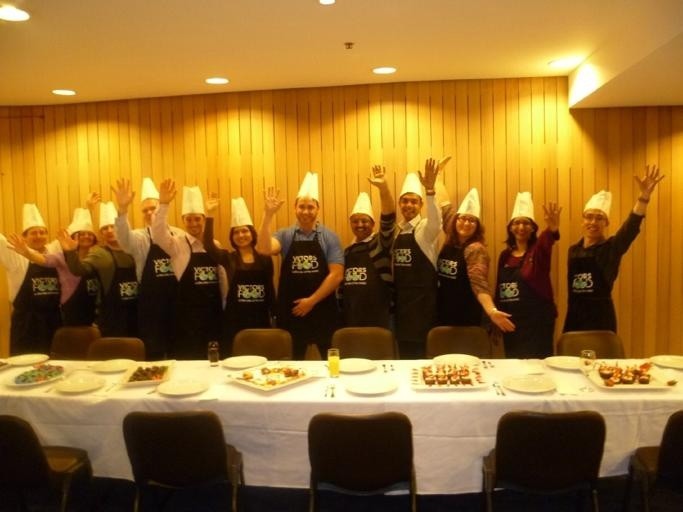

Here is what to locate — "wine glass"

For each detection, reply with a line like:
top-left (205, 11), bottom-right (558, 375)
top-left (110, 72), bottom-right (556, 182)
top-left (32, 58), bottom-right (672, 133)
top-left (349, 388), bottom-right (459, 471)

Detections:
top-left (582, 350), bottom-right (597, 393)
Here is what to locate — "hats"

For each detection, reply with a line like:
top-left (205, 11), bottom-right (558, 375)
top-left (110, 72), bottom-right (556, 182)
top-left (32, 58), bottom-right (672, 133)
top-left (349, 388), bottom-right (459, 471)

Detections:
top-left (350, 192), bottom-right (375, 223)
top-left (511, 192), bottom-right (535, 222)
top-left (182, 186), bottom-right (205, 217)
top-left (23, 204), bottom-right (46, 234)
top-left (583, 190), bottom-right (612, 220)
top-left (99, 201), bottom-right (118, 230)
top-left (141, 177), bottom-right (160, 202)
top-left (457, 187), bottom-right (481, 218)
top-left (399, 173), bottom-right (423, 200)
top-left (231, 198), bottom-right (254, 228)
top-left (67, 208), bottom-right (96, 237)
top-left (296, 172), bottom-right (319, 202)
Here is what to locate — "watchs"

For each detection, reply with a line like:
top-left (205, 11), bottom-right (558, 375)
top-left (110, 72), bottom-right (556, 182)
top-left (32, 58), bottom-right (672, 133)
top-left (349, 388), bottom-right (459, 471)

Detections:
top-left (270, 314), bottom-right (278, 320)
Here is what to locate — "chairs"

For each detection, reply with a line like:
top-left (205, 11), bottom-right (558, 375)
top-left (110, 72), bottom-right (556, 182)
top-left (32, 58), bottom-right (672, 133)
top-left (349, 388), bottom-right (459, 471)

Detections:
top-left (307, 412), bottom-right (416, 512)
top-left (232, 328), bottom-right (293, 361)
top-left (426, 325), bottom-right (492, 359)
top-left (331, 326), bottom-right (400, 360)
top-left (483, 411), bottom-right (606, 512)
top-left (629, 409), bottom-right (683, 512)
top-left (51, 326), bottom-right (101, 360)
top-left (122, 412), bottom-right (245, 512)
top-left (85, 335), bottom-right (146, 361)
top-left (0, 415), bottom-right (92, 512)
top-left (557, 330), bottom-right (626, 359)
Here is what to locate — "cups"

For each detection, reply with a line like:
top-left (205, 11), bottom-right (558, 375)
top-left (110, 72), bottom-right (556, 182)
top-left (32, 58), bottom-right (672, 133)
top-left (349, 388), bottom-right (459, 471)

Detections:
top-left (207, 342), bottom-right (220, 367)
top-left (329, 348), bottom-right (341, 378)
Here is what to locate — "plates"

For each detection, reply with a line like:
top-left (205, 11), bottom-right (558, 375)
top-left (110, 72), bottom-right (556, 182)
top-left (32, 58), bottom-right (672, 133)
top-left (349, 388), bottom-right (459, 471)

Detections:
top-left (156, 375), bottom-right (211, 396)
top-left (53, 370), bottom-right (110, 394)
top-left (119, 359), bottom-right (178, 389)
top-left (223, 356), bottom-right (315, 392)
top-left (502, 353), bottom-right (596, 395)
top-left (588, 354), bottom-right (681, 394)
top-left (1, 354), bottom-right (51, 365)
top-left (2, 360), bottom-right (76, 389)
top-left (325, 355), bottom-right (383, 374)
top-left (220, 354), bottom-right (270, 370)
top-left (88, 357), bottom-right (141, 373)
top-left (344, 369), bottom-right (404, 396)
top-left (409, 356), bottom-right (490, 391)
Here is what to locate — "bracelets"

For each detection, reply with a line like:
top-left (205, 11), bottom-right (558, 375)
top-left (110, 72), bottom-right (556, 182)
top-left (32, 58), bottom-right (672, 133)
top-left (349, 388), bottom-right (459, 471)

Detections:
top-left (28, 249), bottom-right (40, 264)
top-left (637, 196), bottom-right (650, 205)
top-left (425, 189), bottom-right (435, 193)
top-left (487, 308), bottom-right (498, 315)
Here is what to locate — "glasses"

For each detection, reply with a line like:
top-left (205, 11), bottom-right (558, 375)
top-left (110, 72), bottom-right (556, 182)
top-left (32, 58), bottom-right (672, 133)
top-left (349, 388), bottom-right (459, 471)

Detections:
top-left (583, 213), bottom-right (606, 224)
top-left (457, 216), bottom-right (477, 225)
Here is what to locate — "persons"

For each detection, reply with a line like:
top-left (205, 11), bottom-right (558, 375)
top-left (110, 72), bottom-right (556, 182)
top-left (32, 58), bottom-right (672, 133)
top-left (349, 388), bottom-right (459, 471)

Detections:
top-left (490, 191), bottom-right (562, 357)
top-left (202, 192), bottom-right (278, 354)
top-left (152, 178), bottom-right (228, 357)
top-left (2, 189), bottom-right (102, 357)
top-left (7, 209), bottom-right (99, 326)
top-left (256, 172), bottom-right (345, 361)
top-left (391, 157), bottom-right (442, 360)
top-left (111, 177), bottom-right (186, 358)
top-left (337, 165), bottom-right (395, 331)
top-left (56, 200), bottom-right (138, 336)
top-left (433, 155), bottom-right (516, 333)
top-left (557, 164), bottom-right (665, 356)
